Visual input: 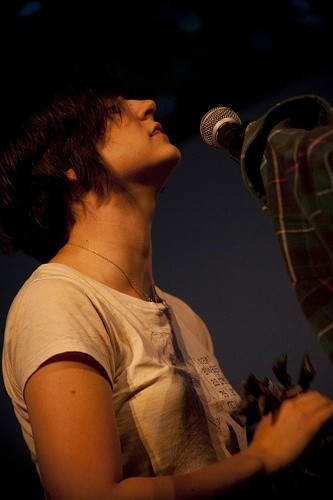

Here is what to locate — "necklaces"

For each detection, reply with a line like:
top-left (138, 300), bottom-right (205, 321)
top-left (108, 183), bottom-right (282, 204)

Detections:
top-left (67, 241), bottom-right (159, 302)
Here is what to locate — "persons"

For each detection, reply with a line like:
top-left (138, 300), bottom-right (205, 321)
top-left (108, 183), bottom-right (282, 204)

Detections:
top-left (0, 76), bottom-right (333, 500)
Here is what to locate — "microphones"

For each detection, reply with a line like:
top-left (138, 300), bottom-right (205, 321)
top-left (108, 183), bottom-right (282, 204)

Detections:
top-left (200, 108), bottom-right (246, 165)
